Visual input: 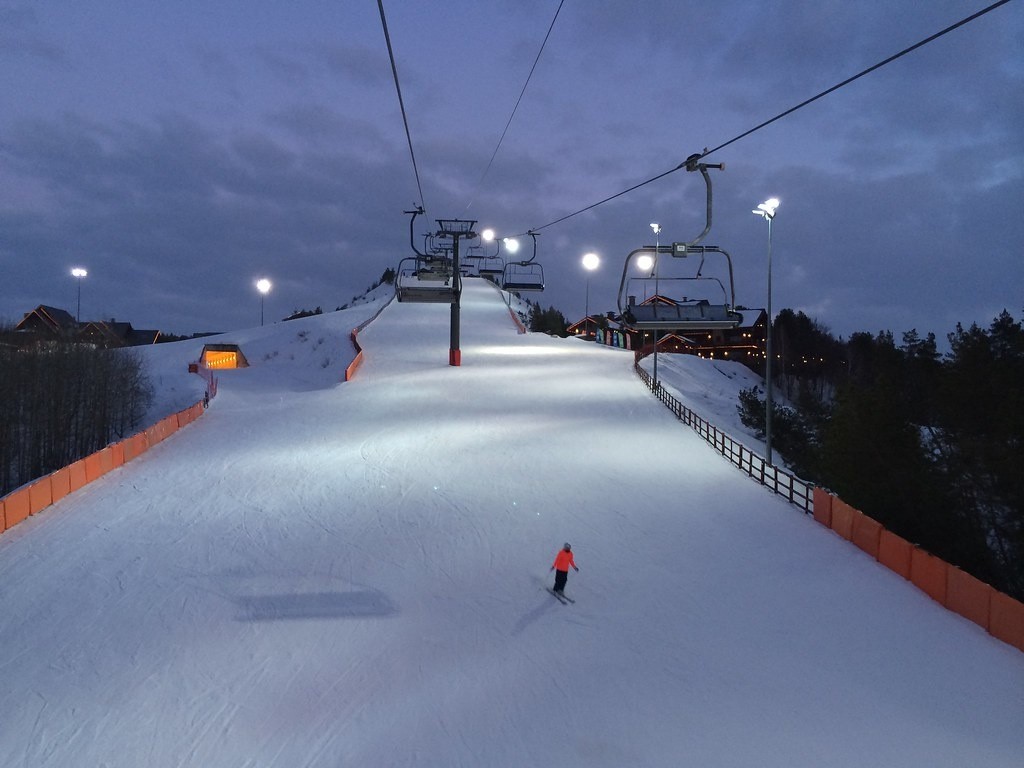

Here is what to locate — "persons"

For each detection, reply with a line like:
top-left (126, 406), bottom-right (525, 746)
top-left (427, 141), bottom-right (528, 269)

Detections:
top-left (205, 391), bottom-right (209, 406)
top-left (549, 542), bottom-right (579, 596)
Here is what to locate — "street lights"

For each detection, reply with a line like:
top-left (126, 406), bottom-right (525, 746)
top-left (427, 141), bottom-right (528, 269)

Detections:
top-left (257, 279), bottom-right (272, 327)
top-left (72, 267), bottom-right (88, 329)
top-left (637, 252), bottom-right (654, 302)
top-left (482, 229), bottom-right (495, 271)
top-left (649, 222), bottom-right (663, 391)
top-left (582, 252), bottom-right (600, 340)
top-left (502, 237), bottom-right (517, 307)
top-left (750, 198), bottom-right (781, 469)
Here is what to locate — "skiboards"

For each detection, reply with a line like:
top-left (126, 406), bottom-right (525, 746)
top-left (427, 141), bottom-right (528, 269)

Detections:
top-left (546, 586), bottom-right (576, 605)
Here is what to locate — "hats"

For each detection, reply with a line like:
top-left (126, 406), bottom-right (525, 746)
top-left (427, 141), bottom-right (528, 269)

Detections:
top-left (565, 542), bottom-right (572, 549)
